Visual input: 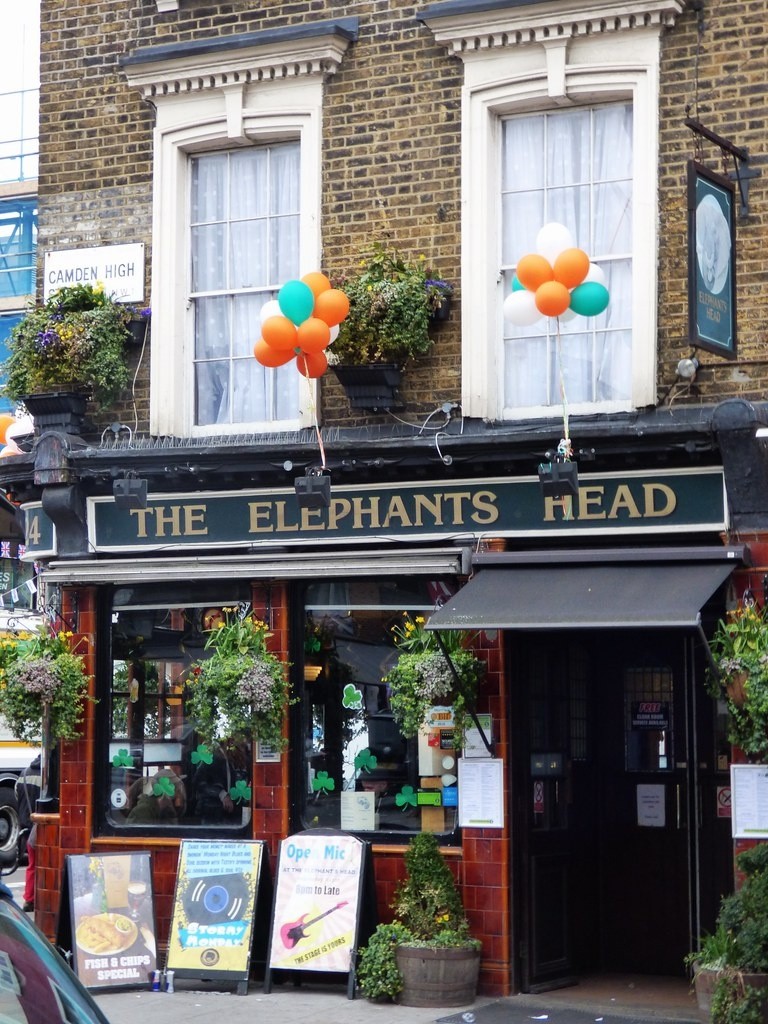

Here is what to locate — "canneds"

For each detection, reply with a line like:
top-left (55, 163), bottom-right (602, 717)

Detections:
top-left (152, 970), bottom-right (161, 992)
top-left (166, 970), bottom-right (175, 993)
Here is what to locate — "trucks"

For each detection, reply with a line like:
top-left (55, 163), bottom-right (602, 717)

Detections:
top-left (0, 700), bottom-right (46, 869)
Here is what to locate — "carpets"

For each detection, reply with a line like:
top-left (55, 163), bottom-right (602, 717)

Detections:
top-left (434, 1000), bottom-right (697, 1024)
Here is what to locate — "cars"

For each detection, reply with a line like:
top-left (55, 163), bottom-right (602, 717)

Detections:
top-left (0, 889), bottom-right (112, 1024)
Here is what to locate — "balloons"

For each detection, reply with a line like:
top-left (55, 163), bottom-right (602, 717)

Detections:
top-left (254, 272), bottom-right (349, 378)
top-left (0, 405), bottom-right (34, 455)
top-left (504, 221), bottom-right (609, 327)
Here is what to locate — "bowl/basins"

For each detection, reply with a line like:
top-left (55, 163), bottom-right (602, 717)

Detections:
top-left (115, 918), bottom-right (136, 933)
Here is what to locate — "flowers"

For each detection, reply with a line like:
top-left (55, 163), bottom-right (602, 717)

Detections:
top-left (0, 283), bottom-right (152, 423)
top-left (0, 615), bottom-right (103, 746)
top-left (380, 611), bottom-right (486, 752)
top-left (323, 243), bottom-right (454, 368)
top-left (355, 907), bottom-right (483, 1001)
top-left (175, 603), bottom-right (301, 755)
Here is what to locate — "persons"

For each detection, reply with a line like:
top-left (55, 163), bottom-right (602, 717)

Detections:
top-left (127, 783), bottom-right (174, 825)
top-left (191, 736), bottom-right (251, 829)
top-left (22, 825), bottom-right (35, 911)
top-left (15, 755), bottom-right (42, 835)
top-left (356, 743), bottom-right (413, 796)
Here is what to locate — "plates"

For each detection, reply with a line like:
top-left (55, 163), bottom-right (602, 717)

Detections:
top-left (76, 913), bottom-right (139, 955)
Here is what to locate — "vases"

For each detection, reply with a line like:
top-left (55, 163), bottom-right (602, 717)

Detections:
top-left (433, 297), bottom-right (450, 322)
top-left (430, 681), bottom-right (457, 706)
top-left (121, 320), bottom-right (145, 346)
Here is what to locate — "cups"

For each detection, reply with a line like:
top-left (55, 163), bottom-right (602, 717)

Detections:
top-left (241, 807), bottom-right (251, 826)
top-left (375, 814), bottom-right (380, 830)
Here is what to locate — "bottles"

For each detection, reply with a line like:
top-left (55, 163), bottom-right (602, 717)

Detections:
top-left (166, 970), bottom-right (175, 993)
top-left (151, 969), bottom-right (162, 991)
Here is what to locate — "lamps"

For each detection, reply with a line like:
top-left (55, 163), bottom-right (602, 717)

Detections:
top-left (539, 450), bottom-right (595, 496)
top-left (10, 432), bottom-right (34, 452)
top-left (113, 469), bottom-right (148, 509)
top-left (295, 466), bottom-right (331, 508)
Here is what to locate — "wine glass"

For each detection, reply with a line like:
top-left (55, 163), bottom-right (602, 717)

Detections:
top-left (126, 880), bottom-right (146, 922)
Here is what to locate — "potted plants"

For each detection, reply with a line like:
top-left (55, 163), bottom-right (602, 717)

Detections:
top-left (684, 842), bottom-right (767, 1023)
top-left (387, 838), bottom-right (480, 1007)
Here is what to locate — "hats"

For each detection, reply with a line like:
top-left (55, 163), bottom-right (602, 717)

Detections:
top-left (148, 769), bottom-right (181, 797)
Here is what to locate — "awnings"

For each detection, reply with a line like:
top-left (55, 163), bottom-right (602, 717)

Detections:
top-left (424, 568), bottom-right (747, 752)
